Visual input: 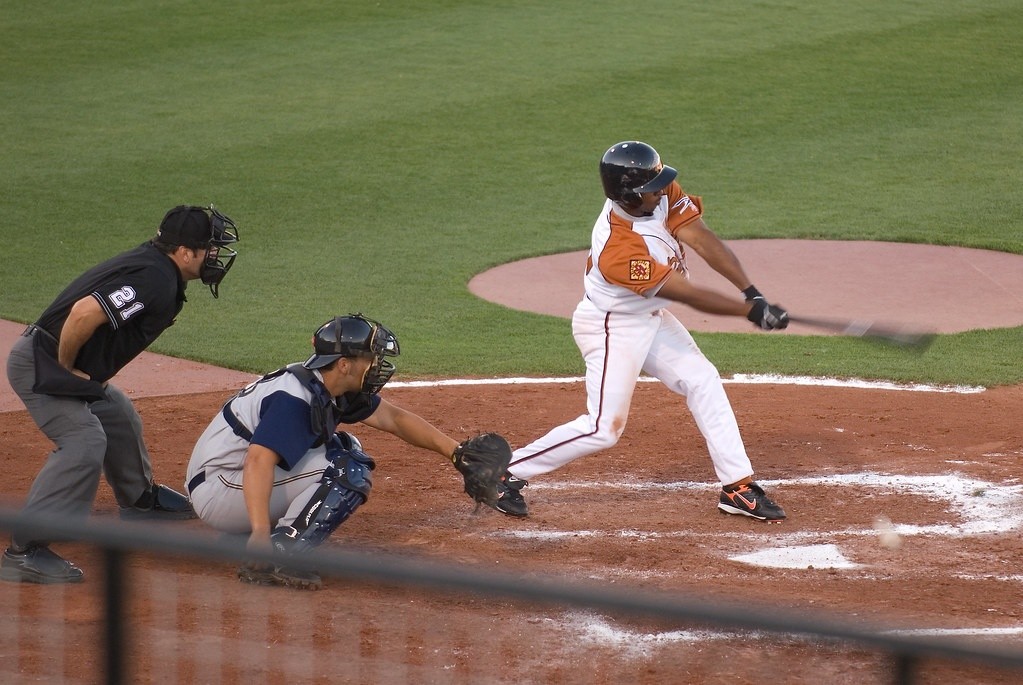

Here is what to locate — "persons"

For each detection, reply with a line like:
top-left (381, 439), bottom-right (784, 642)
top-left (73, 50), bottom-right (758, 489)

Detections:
top-left (497, 140), bottom-right (789, 524)
top-left (184, 311), bottom-right (528, 591)
top-left (0, 203), bottom-right (239, 584)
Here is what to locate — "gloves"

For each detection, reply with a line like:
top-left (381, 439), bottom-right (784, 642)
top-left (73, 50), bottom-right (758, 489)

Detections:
top-left (744, 298), bottom-right (789, 331)
top-left (741, 286), bottom-right (764, 308)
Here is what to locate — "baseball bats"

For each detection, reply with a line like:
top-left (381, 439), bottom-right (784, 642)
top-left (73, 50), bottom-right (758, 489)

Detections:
top-left (786, 311), bottom-right (937, 357)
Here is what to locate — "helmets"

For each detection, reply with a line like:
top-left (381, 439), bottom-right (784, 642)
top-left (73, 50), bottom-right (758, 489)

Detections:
top-left (302, 313), bottom-right (375, 369)
top-left (599, 141), bottom-right (678, 212)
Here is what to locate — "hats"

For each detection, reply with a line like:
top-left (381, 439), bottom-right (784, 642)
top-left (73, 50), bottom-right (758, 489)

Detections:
top-left (157, 205), bottom-right (212, 248)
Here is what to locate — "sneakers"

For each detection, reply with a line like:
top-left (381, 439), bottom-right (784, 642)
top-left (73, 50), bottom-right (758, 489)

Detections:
top-left (717, 481), bottom-right (787, 525)
top-left (0, 545), bottom-right (85, 584)
top-left (492, 470), bottom-right (530, 517)
top-left (117, 484), bottom-right (198, 521)
top-left (239, 566), bottom-right (324, 591)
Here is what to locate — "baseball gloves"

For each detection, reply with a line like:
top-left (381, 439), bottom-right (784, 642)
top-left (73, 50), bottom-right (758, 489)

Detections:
top-left (450, 432), bottom-right (528, 520)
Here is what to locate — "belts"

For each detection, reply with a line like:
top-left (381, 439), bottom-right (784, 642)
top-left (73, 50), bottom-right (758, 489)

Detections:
top-left (27, 326), bottom-right (37, 335)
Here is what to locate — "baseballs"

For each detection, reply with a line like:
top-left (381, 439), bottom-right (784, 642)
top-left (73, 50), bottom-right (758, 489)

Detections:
top-left (873, 513), bottom-right (903, 549)
